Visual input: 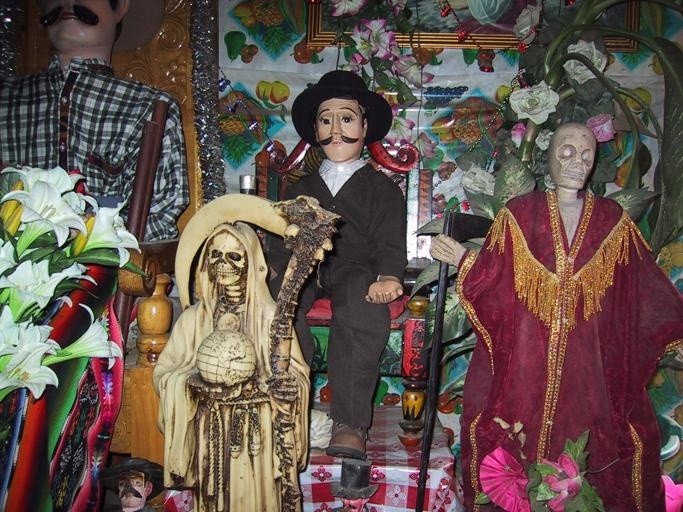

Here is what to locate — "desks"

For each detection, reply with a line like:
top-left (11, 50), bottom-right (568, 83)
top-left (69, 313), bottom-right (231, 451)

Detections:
top-left (156, 400), bottom-right (457, 511)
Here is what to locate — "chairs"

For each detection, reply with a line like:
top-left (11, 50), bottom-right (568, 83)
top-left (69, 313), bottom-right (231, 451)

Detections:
top-left (254, 129), bottom-right (436, 452)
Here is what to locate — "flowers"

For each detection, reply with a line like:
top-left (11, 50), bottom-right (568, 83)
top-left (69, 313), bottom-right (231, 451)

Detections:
top-left (1, 158), bottom-right (152, 403)
top-left (501, 1), bottom-right (683, 262)
top-left (328, 1), bottom-right (437, 150)
top-left (535, 429), bottom-right (606, 511)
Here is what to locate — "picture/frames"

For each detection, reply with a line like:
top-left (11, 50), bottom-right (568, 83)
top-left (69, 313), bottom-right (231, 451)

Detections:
top-left (304, 1), bottom-right (643, 58)
